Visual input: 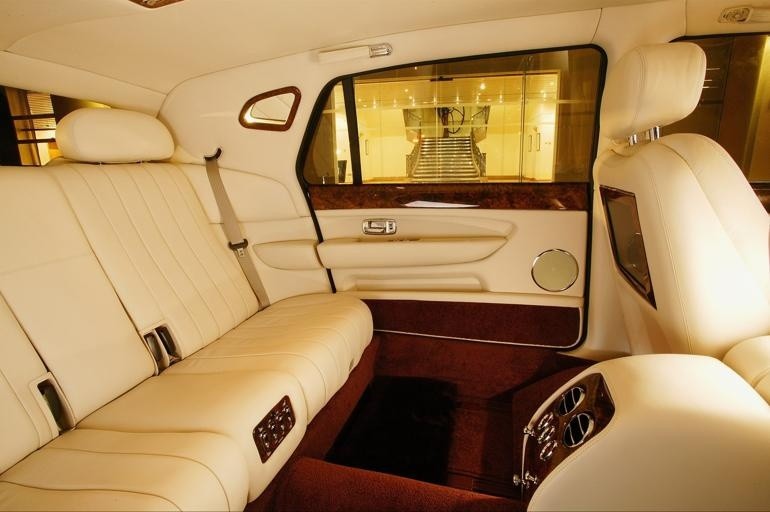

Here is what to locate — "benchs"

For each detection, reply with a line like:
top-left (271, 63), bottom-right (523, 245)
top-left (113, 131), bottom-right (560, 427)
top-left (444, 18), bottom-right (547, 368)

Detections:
top-left (0, 106), bottom-right (374, 512)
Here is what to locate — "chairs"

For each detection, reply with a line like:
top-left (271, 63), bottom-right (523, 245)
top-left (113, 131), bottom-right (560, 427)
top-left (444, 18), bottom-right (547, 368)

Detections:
top-left (593, 42), bottom-right (770, 357)
